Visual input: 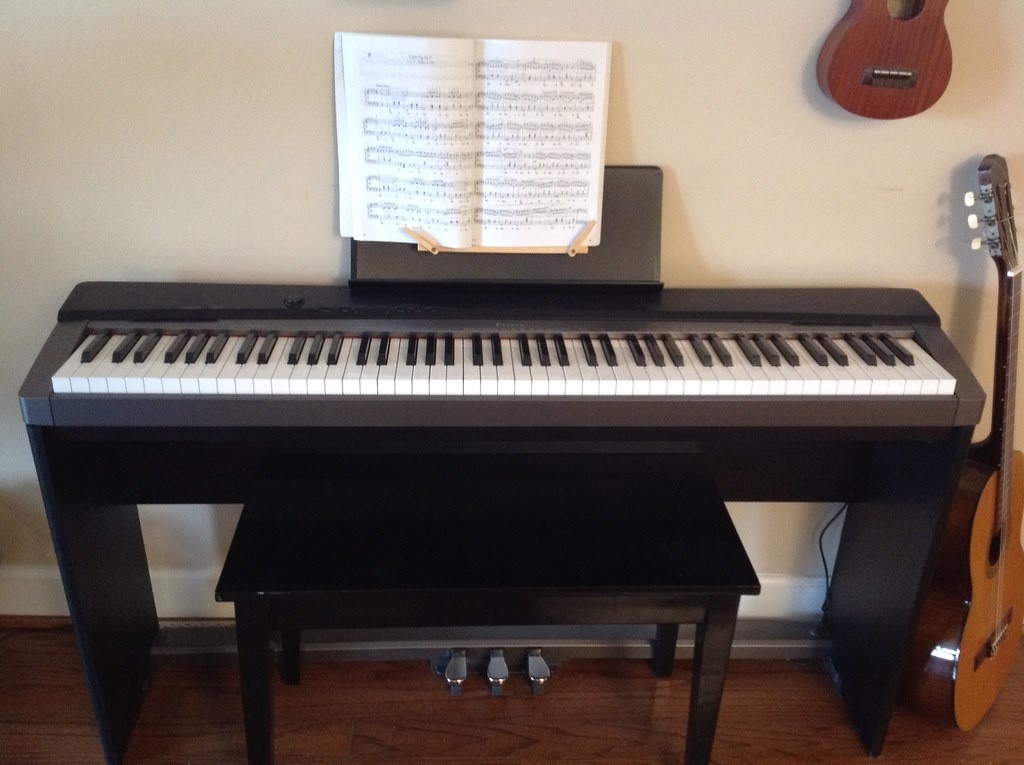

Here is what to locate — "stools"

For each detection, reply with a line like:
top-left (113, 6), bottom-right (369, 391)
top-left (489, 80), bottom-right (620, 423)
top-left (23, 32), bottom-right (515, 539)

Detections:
top-left (215, 458), bottom-right (760, 765)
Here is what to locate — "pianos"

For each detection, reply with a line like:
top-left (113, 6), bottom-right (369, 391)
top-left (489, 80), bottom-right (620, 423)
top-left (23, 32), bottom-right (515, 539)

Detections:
top-left (18, 279), bottom-right (991, 765)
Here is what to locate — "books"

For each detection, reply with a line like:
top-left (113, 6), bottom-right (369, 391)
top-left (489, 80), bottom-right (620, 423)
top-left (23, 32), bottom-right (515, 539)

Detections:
top-left (333, 32), bottom-right (613, 249)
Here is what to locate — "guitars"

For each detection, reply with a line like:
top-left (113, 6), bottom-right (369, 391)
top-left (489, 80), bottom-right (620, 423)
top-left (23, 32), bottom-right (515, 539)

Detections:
top-left (817, 0), bottom-right (955, 121)
top-left (902, 154), bottom-right (1024, 735)
